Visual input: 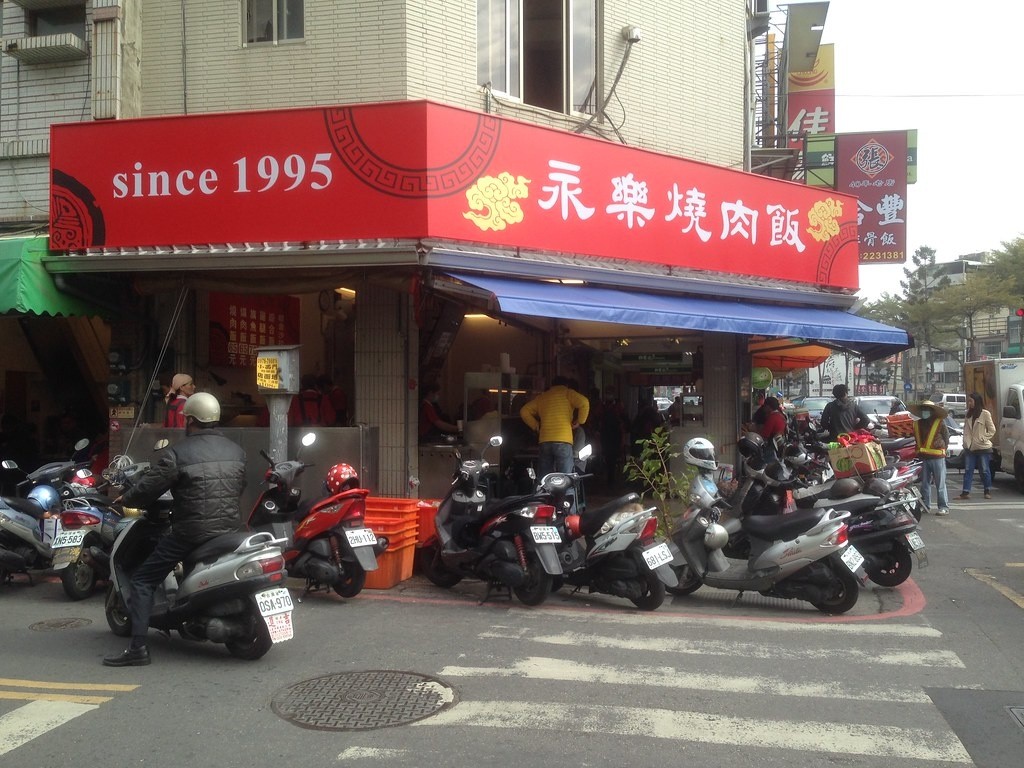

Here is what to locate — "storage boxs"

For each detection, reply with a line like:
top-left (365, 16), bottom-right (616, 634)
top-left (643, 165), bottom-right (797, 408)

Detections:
top-left (828, 442), bottom-right (886, 478)
top-left (365, 497), bottom-right (442, 589)
top-left (885, 414), bottom-right (914, 438)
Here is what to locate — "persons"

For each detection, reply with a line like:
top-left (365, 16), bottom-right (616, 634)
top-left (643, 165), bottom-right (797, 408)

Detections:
top-left (160, 369), bottom-right (349, 430)
top-left (953, 393), bottom-right (996, 500)
top-left (890, 399), bottom-right (900, 415)
top-left (742, 388), bottom-right (788, 439)
top-left (906, 400), bottom-right (950, 516)
top-left (419, 376), bottom-right (680, 514)
top-left (104, 392), bottom-right (248, 666)
top-left (821, 384), bottom-right (870, 442)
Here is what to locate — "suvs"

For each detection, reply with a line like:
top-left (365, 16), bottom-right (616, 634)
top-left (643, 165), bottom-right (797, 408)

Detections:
top-left (851, 395), bottom-right (922, 436)
top-left (802, 396), bottom-right (834, 430)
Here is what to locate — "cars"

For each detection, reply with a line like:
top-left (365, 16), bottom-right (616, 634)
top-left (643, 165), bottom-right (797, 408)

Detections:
top-left (942, 417), bottom-right (966, 468)
top-left (654, 397), bottom-right (674, 411)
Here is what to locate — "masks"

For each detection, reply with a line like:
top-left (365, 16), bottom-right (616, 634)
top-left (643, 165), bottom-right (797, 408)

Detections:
top-left (921, 411), bottom-right (930, 420)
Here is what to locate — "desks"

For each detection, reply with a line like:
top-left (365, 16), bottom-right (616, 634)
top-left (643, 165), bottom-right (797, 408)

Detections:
top-left (512, 453), bottom-right (596, 515)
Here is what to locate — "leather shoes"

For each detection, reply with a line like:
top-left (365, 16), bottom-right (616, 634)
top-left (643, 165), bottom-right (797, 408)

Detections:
top-left (103, 644), bottom-right (151, 666)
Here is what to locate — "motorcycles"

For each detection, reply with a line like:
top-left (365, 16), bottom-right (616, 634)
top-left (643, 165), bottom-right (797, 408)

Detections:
top-left (51, 437), bottom-right (169, 601)
top-left (0, 436), bottom-right (90, 593)
top-left (247, 431), bottom-right (388, 602)
top-left (660, 439), bottom-right (869, 613)
top-left (97, 438), bottom-right (295, 663)
top-left (731, 420), bottom-right (926, 589)
top-left (526, 442), bottom-right (678, 611)
top-left (432, 440), bottom-right (564, 607)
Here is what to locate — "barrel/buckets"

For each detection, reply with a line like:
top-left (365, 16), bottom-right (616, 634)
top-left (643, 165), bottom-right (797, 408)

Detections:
top-left (416, 499), bottom-right (444, 544)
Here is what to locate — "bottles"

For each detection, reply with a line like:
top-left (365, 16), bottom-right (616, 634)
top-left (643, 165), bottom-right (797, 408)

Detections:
top-left (690, 383), bottom-right (694, 393)
top-left (683, 383), bottom-right (687, 392)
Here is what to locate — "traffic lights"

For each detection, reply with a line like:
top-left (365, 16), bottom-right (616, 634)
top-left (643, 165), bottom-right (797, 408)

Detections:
top-left (1015, 309), bottom-right (1024, 316)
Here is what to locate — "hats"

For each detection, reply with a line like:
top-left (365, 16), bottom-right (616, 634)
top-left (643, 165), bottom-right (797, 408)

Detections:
top-left (907, 400), bottom-right (948, 419)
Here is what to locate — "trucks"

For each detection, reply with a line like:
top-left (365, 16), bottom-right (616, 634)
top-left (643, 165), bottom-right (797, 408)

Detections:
top-left (963, 356), bottom-right (1024, 496)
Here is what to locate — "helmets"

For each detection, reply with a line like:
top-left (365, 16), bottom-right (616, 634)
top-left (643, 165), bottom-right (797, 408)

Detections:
top-left (683, 437), bottom-right (718, 470)
top-left (27, 485), bottom-right (61, 511)
top-left (829, 478), bottom-right (859, 498)
top-left (737, 432), bottom-right (765, 459)
top-left (178, 392), bottom-right (221, 422)
top-left (326, 462), bottom-right (359, 494)
top-left (863, 478), bottom-right (893, 498)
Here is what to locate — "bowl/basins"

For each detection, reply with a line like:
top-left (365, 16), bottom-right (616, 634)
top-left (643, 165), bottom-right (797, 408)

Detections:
top-left (480, 353), bottom-right (517, 373)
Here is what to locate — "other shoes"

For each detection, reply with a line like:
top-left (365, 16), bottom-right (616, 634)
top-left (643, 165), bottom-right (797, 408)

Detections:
top-left (984, 494), bottom-right (991, 499)
top-left (952, 492), bottom-right (970, 500)
top-left (936, 508), bottom-right (950, 516)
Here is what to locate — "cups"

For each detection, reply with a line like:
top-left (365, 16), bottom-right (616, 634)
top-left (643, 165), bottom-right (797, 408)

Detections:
top-left (457, 420), bottom-right (463, 432)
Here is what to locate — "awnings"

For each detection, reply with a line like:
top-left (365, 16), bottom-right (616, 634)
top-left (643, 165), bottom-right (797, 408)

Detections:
top-left (442, 272), bottom-right (915, 361)
top-left (0, 236), bottom-right (117, 321)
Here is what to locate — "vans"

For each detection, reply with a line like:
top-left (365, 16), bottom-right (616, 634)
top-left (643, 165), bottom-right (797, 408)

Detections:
top-left (929, 394), bottom-right (967, 418)
